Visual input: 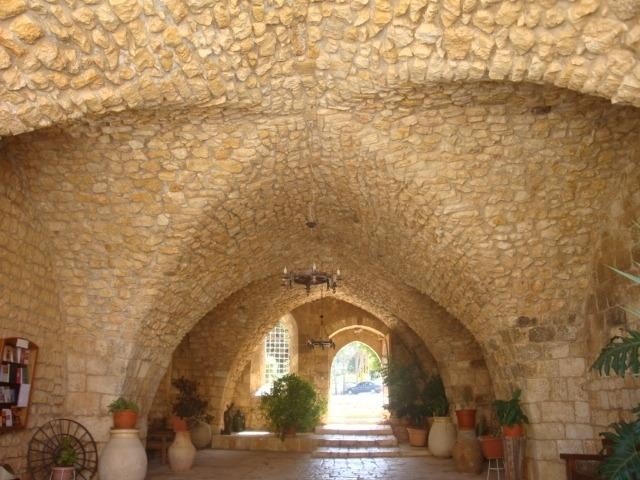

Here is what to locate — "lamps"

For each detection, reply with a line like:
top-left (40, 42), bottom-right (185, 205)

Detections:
top-left (306, 300), bottom-right (336, 349)
top-left (281, 219), bottom-right (342, 298)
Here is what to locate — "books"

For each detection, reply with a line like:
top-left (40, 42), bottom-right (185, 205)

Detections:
top-left (0, 344), bottom-right (31, 429)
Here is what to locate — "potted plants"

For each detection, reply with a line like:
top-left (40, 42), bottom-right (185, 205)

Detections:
top-left (377, 355), bottom-right (529, 479)
top-left (259, 374), bottom-right (328, 440)
top-left (108, 396), bottom-right (140, 429)
top-left (48, 438), bottom-right (77, 480)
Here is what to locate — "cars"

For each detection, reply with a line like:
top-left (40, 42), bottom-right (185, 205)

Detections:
top-left (347, 381), bottom-right (382, 395)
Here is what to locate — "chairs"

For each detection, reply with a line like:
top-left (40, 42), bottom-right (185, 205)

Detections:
top-left (147, 411), bottom-right (176, 465)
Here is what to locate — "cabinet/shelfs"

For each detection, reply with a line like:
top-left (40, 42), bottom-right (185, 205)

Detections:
top-left (1, 338), bottom-right (39, 435)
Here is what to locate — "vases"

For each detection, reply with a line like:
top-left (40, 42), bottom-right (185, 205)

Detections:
top-left (95, 430), bottom-right (148, 480)
top-left (166, 430), bottom-right (195, 472)
